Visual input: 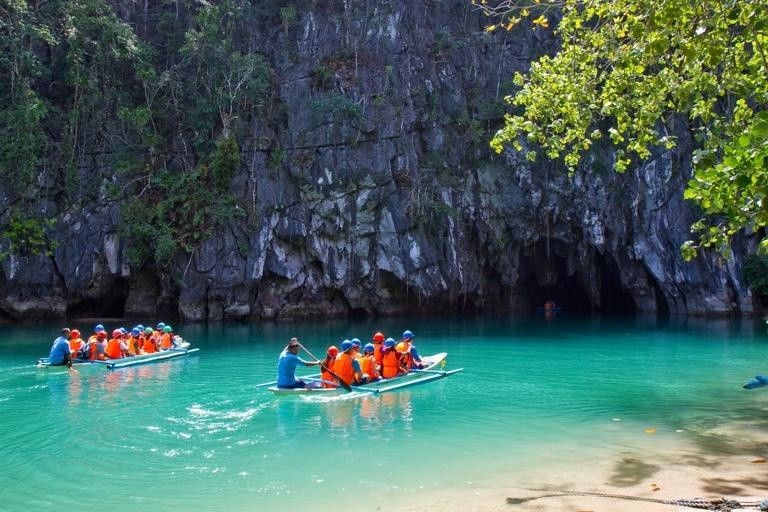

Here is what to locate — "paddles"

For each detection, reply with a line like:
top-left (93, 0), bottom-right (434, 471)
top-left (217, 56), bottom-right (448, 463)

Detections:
top-left (299, 343), bottom-right (352, 392)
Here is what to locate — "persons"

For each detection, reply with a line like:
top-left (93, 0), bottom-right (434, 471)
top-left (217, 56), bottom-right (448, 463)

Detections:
top-left (49, 321), bottom-right (179, 366)
top-left (321, 329), bottom-right (421, 387)
top-left (278, 391), bottom-right (411, 436)
top-left (48, 364), bottom-right (170, 411)
top-left (277, 337), bottom-right (321, 388)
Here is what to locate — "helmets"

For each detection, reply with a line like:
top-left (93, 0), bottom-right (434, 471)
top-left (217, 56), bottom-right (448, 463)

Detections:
top-left (98, 330), bottom-right (108, 339)
top-left (363, 342), bottom-right (375, 353)
top-left (131, 328), bottom-right (141, 338)
top-left (136, 324), bottom-right (144, 331)
top-left (119, 327), bottom-right (128, 336)
top-left (157, 322), bottom-right (166, 330)
top-left (341, 340), bottom-right (355, 353)
top-left (372, 332), bottom-right (385, 341)
top-left (70, 329), bottom-right (81, 339)
top-left (351, 337), bottom-right (362, 348)
top-left (112, 328), bottom-right (124, 339)
top-left (384, 337), bottom-right (396, 348)
top-left (163, 325), bottom-right (174, 333)
top-left (326, 346), bottom-right (338, 358)
top-left (95, 324), bottom-right (104, 334)
top-left (402, 329), bottom-right (416, 341)
top-left (145, 326), bottom-right (153, 336)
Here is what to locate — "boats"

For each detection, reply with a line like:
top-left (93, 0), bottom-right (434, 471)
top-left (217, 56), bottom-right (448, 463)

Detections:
top-left (255, 349), bottom-right (463, 401)
top-left (534, 307), bottom-right (562, 312)
top-left (35, 336), bottom-right (203, 370)
